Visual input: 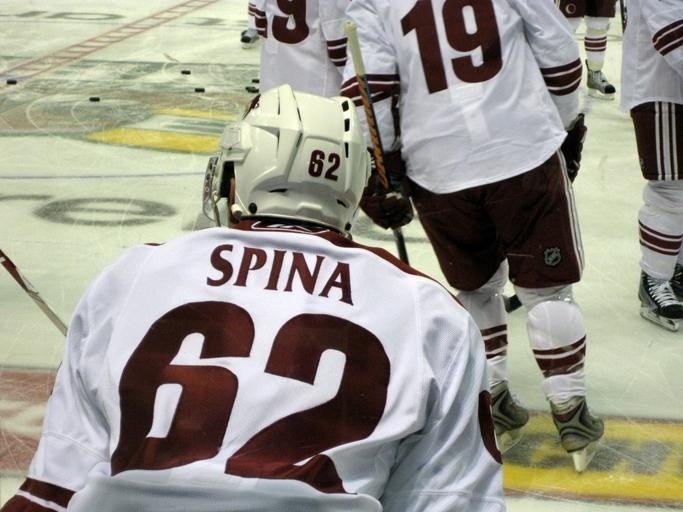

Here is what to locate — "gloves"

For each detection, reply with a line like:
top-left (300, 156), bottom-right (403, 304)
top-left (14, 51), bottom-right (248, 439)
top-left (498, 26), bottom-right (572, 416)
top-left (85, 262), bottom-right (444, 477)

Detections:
top-left (358, 147), bottom-right (413, 228)
top-left (562, 113), bottom-right (586, 181)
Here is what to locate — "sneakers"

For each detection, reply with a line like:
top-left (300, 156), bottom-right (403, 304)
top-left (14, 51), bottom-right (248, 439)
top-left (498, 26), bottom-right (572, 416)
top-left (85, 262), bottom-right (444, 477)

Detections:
top-left (639, 264), bottom-right (683, 318)
top-left (550, 396), bottom-right (603, 452)
top-left (490, 381), bottom-right (528, 434)
top-left (588, 71), bottom-right (614, 93)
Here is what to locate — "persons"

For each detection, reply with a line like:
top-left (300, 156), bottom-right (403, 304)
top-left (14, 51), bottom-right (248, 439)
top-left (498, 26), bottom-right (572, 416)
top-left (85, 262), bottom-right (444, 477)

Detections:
top-left (242, 0), bottom-right (682, 475)
top-left (1, 82), bottom-right (504, 512)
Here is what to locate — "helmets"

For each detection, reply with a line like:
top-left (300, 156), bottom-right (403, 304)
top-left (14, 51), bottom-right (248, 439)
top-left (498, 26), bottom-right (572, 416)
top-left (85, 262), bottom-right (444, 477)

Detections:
top-left (203, 85), bottom-right (372, 236)
